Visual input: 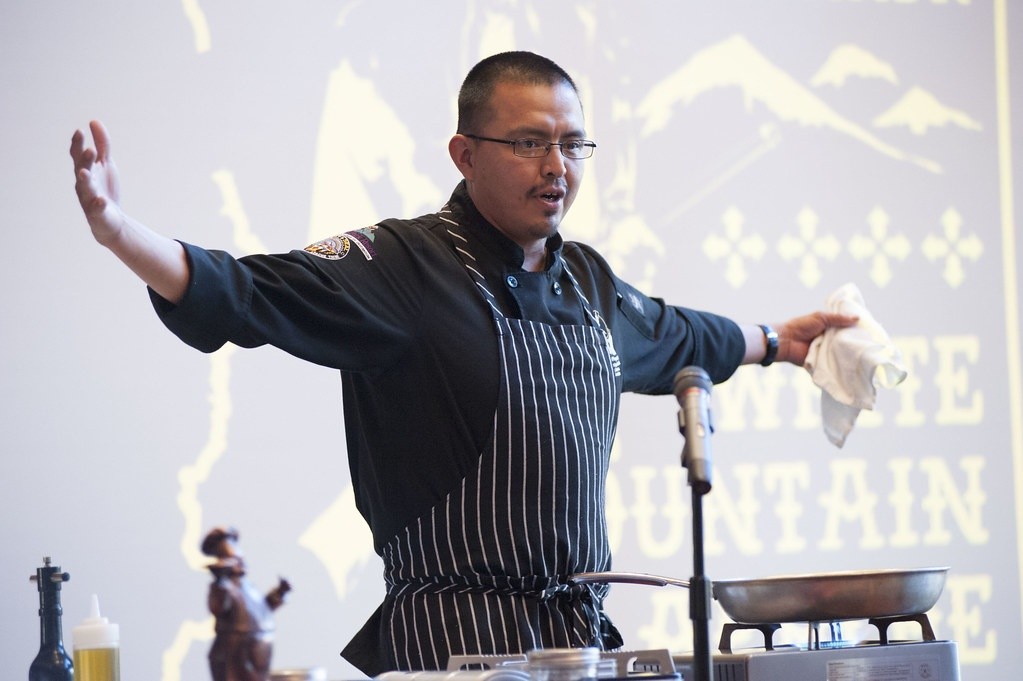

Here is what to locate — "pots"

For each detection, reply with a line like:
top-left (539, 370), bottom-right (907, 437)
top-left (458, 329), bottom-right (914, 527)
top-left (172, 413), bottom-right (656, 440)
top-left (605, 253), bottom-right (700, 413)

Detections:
top-left (565, 567), bottom-right (952, 625)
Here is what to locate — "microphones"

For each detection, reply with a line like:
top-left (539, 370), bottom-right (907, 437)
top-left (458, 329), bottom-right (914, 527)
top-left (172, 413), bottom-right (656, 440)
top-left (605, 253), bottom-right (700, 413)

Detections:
top-left (672, 365), bottom-right (715, 495)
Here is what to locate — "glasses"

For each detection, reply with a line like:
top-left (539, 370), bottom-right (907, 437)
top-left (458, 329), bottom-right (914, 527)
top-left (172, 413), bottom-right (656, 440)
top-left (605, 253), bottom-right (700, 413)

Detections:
top-left (464, 134), bottom-right (597, 159)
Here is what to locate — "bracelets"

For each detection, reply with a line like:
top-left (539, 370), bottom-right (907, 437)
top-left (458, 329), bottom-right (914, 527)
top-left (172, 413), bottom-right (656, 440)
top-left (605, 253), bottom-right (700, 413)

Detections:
top-left (761, 326), bottom-right (778, 366)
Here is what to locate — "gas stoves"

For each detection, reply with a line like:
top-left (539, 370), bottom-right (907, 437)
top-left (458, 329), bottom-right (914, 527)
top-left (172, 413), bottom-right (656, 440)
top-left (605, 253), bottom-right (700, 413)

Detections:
top-left (673, 614), bottom-right (959, 681)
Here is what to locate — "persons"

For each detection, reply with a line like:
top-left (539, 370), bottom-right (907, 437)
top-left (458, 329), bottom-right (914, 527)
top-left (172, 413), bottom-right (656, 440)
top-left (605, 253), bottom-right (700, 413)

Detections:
top-left (70, 51), bottom-right (860, 681)
top-left (203, 526), bottom-right (291, 680)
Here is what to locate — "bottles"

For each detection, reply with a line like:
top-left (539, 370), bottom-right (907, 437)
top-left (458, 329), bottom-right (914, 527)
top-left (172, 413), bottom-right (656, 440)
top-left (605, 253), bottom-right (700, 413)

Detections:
top-left (72, 595), bottom-right (121, 681)
